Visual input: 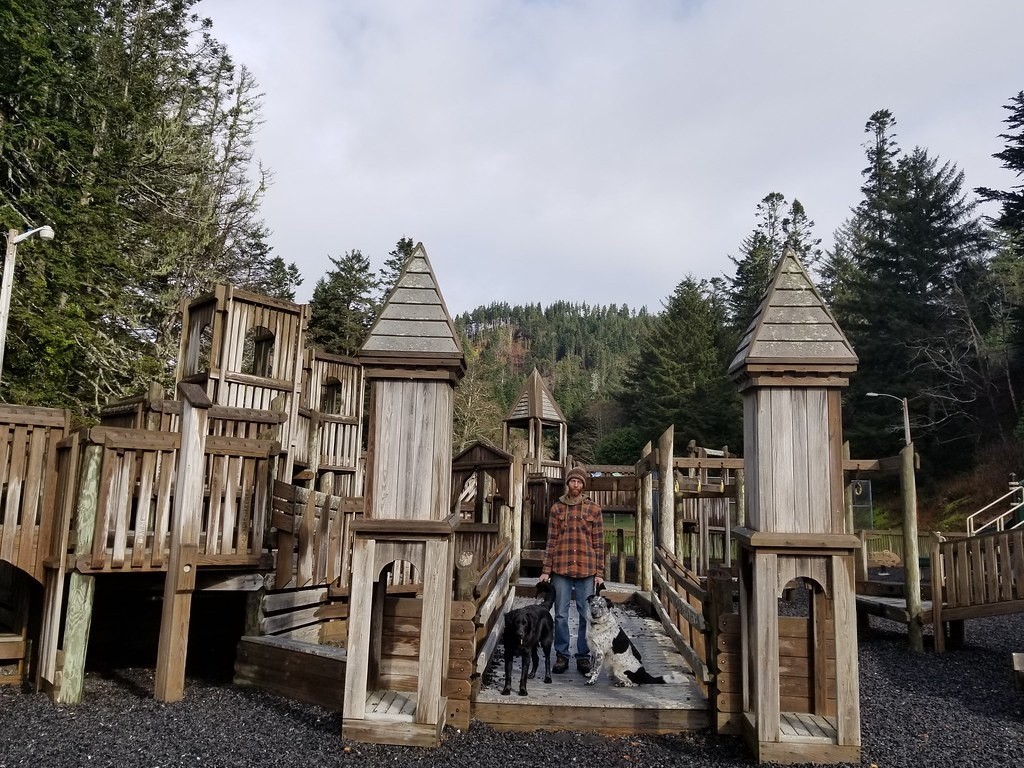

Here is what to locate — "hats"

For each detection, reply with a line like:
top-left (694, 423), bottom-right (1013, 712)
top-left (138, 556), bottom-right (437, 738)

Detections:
top-left (566, 467), bottom-right (587, 488)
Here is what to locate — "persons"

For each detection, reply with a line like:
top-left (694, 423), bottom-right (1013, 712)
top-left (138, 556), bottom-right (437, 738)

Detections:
top-left (538, 467), bottom-right (604, 675)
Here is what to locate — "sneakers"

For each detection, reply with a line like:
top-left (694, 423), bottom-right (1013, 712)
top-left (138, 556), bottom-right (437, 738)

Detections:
top-left (577, 659), bottom-right (591, 674)
top-left (553, 656), bottom-right (569, 673)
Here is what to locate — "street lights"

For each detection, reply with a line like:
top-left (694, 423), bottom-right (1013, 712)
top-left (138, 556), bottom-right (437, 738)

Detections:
top-left (0, 225), bottom-right (54, 376)
top-left (866, 393), bottom-right (912, 446)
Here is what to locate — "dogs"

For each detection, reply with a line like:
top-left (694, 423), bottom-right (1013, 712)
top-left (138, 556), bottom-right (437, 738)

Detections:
top-left (501, 579), bottom-right (558, 696)
top-left (583, 593), bottom-right (690, 687)
